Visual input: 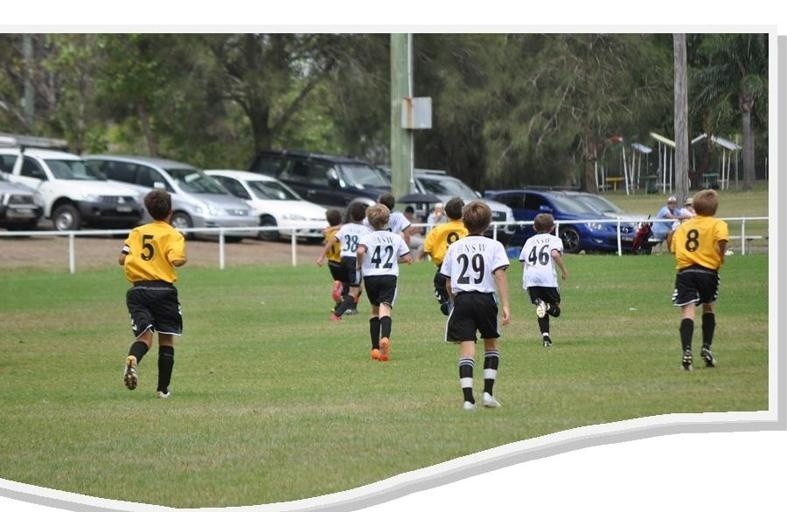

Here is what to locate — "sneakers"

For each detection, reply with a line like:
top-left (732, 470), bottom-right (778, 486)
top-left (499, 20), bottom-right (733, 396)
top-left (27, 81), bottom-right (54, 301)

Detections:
top-left (541, 335), bottom-right (553, 349)
top-left (327, 313), bottom-right (343, 321)
top-left (682, 351), bottom-right (695, 371)
top-left (378, 337), bottom-right (390, 361)
top-left (156, 390), bottom-right (171, 400)
top-left (332, 280), bottom-right (343, 302)
top-left (700, 348), bottom-right (718, 368)
top-left (481, 391), bottom-right (502, 408)
top-left (371, 348), bottom-right (380, 359)
top-left (123, 354), bottom-right (139, 390)
top-left (344, 308), bottom-right (359, 315)
top-left (533, 296), bottom-right (549, 320)
top-left (463, 401), bottom-right (478, 412)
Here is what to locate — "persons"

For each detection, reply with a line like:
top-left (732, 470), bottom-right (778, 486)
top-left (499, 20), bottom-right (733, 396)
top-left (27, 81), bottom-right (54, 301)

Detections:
top-left (118, 189), bottom-right (187, 399)
top-left (671, 197), bottom-right (697, 230)
top-left (356, 204), bottom-right (412, 361)
top-left (403, 204), bottom-right (426, 264)
top-left (519, 214), bottom-right (569, 347)
top-left (650, 197), bottom-right (681, 254)
top-left (316, 200), bottom-right (372, 321)
top-left (323, 210), bottom-right (358, 315)
top-left (670, 189), bottom-right (729, 371)
top-left (425, 202), bottom-right (454, 246)
top-left (439, 200), bottom-right (510, 411)
top-left (415, 196), bottom-right (470, 315)
top-left (379, 194), bottom-right (413, 245)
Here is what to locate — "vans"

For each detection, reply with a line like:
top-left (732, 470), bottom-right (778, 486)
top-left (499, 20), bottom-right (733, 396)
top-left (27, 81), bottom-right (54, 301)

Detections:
top-left (80, 151), bottom-right (261, 243)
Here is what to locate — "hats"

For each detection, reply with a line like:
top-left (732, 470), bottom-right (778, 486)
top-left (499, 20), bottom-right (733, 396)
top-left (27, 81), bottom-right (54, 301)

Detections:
top-left (667, 196), bottom-right (677, 204)
top-left (684, 197), bottom-right (693, 206)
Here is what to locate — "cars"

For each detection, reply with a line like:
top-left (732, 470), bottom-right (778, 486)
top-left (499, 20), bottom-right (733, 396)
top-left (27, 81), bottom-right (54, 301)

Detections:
top-left (204, 167), bottom-right (329, 242)
top-left (486, 184), bottom-right (636, 254)
top-left (412, 169), bottom-right (516, 246)
top-left (0, 151), bottom-right (45, 229)
top-left (0, 128), bottom-right (140, 231)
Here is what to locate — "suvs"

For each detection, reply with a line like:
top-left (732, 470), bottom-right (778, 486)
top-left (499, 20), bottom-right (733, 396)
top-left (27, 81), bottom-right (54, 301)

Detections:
top-left (275, 145), bottom-right (398, 212)
